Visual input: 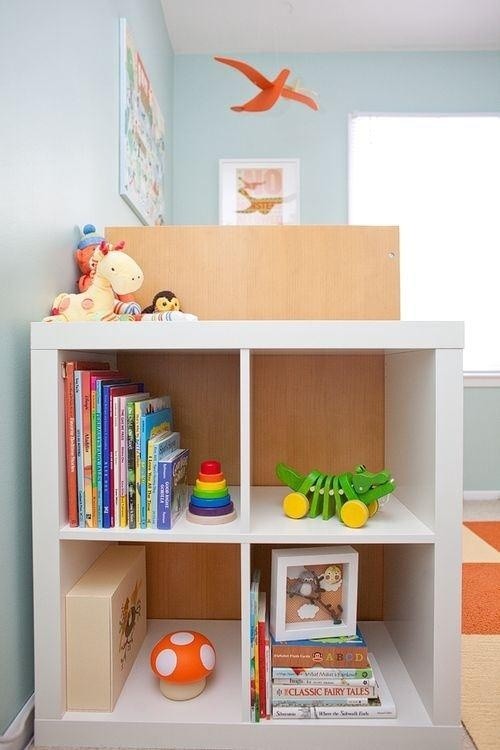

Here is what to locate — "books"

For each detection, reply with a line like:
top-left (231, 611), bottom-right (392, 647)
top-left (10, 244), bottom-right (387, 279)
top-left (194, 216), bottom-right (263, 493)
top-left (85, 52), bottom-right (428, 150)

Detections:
top-left (61, 360), bottom-right (190, 530)
top-left (249, 570), bottom-right (398, 723)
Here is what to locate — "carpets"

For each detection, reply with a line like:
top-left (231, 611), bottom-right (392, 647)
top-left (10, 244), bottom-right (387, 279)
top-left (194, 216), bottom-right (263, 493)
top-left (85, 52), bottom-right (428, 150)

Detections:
top-left (462, 521), bottom-right (500, 634)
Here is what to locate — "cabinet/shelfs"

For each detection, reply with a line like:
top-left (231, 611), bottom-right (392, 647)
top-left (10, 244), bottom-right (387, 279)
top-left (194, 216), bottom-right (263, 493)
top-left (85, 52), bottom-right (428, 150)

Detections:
top-left (28, 319), bottom-right (464, 750)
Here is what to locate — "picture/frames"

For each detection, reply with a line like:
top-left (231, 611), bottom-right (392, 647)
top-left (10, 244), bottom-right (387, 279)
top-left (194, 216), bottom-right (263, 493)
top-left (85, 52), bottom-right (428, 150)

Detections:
top-left (268, 545), bottom-right (360, 643)
top-left (218, 158), bottom-right (300, 226)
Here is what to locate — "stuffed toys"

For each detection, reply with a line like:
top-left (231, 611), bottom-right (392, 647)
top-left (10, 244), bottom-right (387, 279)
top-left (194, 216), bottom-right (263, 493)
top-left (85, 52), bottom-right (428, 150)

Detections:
top-left (41, 223), bottom-right (198, 321)
